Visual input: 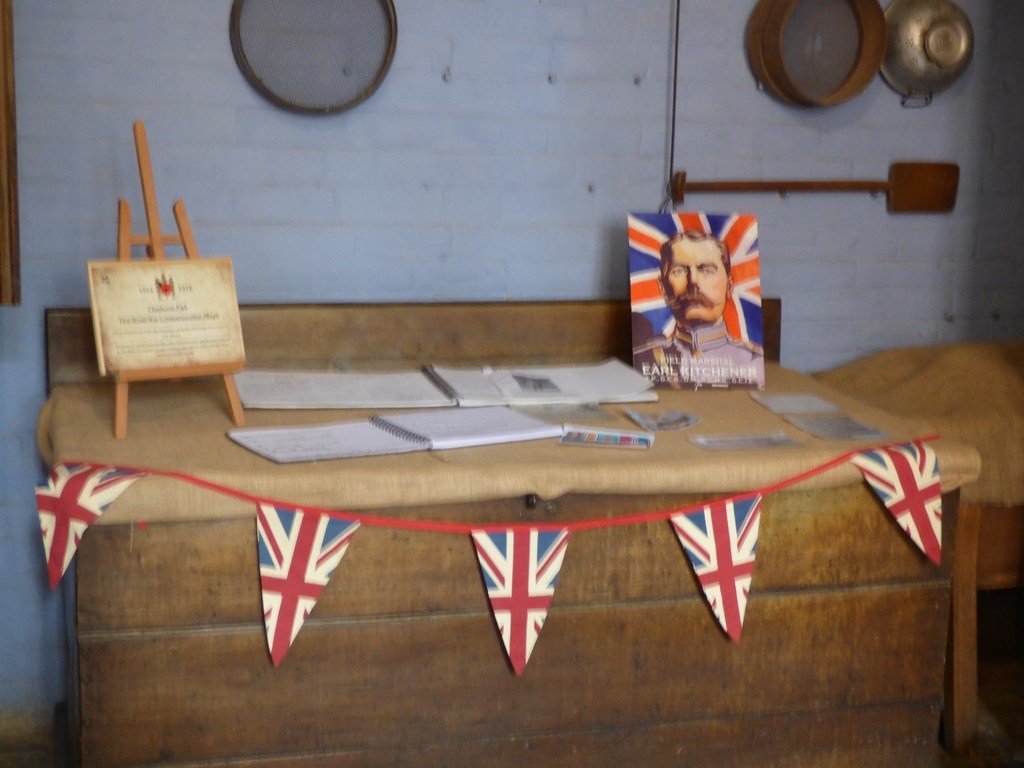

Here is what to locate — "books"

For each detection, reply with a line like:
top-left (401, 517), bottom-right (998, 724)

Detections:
top-left (234, 358), bottom-right (659, 410)
top-left (228, 405), bottom-right (564, 462)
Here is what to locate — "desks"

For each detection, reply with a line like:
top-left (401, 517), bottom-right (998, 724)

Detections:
top-left (945, 487), bottom-right (1024, 756)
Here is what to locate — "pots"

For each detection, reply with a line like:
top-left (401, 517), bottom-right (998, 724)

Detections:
top-left (879, 0), bottom-right (975, 109)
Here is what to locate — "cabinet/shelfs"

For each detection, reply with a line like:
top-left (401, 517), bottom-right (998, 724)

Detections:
top-left (44, 298), bottom-right (983, 768)
